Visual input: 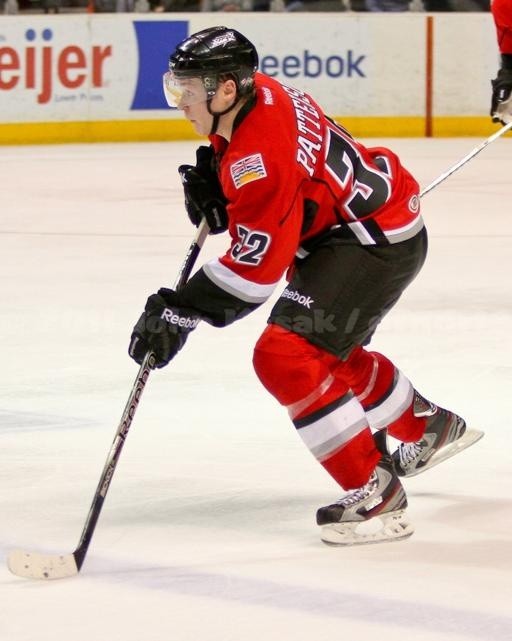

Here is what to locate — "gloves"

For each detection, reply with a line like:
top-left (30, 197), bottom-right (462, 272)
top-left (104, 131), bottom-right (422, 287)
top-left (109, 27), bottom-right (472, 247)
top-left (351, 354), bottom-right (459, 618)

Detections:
top-left (127, 286), bottom-right (203, 369)
top-left (490, 52), bottom-right (511, 136)
top-left (177, 144), bottom-right (230, 236)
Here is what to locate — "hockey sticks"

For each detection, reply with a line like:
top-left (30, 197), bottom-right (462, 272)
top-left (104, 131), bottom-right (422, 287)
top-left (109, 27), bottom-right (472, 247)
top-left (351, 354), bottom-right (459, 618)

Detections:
top-left (8, 214), bottom-right (211, 580)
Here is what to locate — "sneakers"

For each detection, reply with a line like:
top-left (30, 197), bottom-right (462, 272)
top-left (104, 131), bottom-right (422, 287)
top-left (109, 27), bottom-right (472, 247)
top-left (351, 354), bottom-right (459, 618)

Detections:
top-left (391, 397), bottom-right (465, 478)
top-left (314, 444), bottom-right (408, 527)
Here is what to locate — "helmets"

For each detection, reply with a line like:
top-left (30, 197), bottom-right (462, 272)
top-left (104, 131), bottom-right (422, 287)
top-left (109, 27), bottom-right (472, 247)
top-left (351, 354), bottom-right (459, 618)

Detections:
top-left (162, 25), bottom-right (260, 108)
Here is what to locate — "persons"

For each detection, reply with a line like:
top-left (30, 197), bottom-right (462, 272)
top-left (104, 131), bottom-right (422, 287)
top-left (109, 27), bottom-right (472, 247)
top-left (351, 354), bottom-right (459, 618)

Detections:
top-left (144, 27), bottom-right (466, 526)
top-left (491, 0), bottom-right (512, 116)
